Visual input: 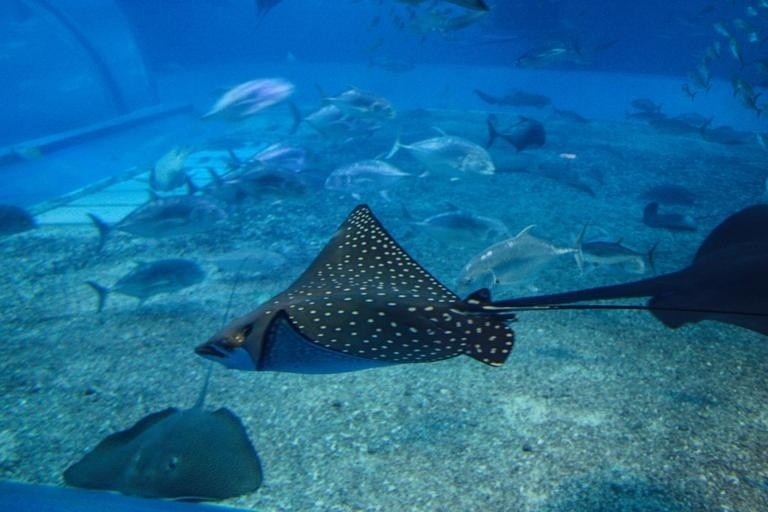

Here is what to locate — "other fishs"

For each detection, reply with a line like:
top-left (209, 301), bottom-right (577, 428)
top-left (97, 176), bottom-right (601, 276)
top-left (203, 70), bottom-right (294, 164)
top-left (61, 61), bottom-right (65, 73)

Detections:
top-left (82, 70), bottom-right (584, 313)
top-left (487, 192), bottom-right (768, 345)
